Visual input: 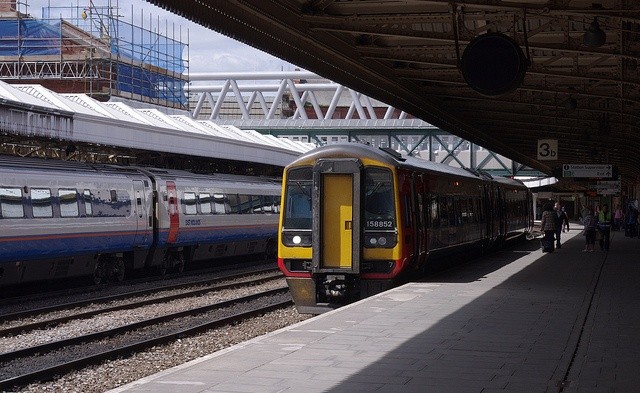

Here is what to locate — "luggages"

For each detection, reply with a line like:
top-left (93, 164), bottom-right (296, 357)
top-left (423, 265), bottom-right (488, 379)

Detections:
top-left (541, 230), bottom-right (553, 253)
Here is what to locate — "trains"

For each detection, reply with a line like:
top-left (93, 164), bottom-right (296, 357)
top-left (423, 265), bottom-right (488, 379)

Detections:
top-left (278, 139), bottom-right (535, 314)
top-left (0, 152), bottom-right (284, 292)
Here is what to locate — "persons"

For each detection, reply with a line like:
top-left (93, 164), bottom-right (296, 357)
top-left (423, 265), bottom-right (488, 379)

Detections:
top-left (560, 203), bottom-right (567, 233)
top-left (553, 202), bottom-right (569, 249)
top-left (579, 203), bottom-right (640, 254)
top-left (540, 202), bottom-right (558, 254)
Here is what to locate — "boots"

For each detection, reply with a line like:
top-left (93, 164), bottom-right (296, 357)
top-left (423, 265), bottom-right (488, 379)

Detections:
top-left (589, 244), bottom-right (594, 251)
top-left (582, 245), bottom-right (589, 252)
top-left (600, 240), bottom-right (604, 250)
top-left (604, 239), bottom-right (610, 250)
